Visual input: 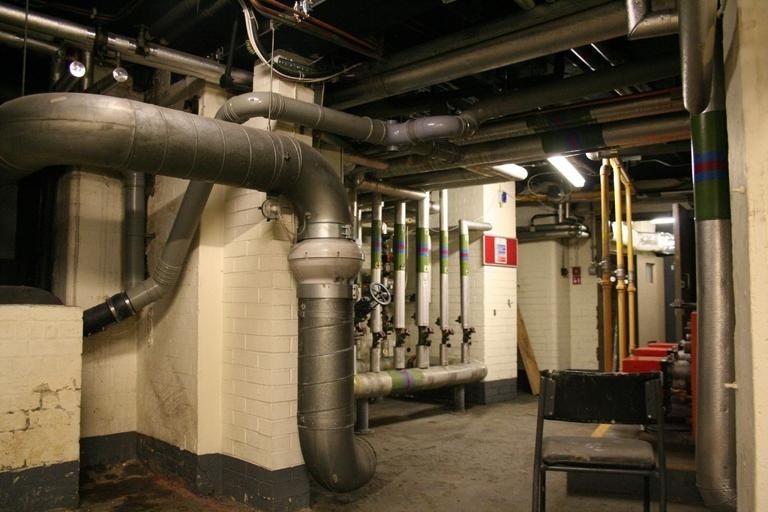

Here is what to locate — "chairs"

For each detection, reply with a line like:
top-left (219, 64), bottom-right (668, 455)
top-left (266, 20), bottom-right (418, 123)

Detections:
top-left (532, 368), bottom-right (667, 511)
